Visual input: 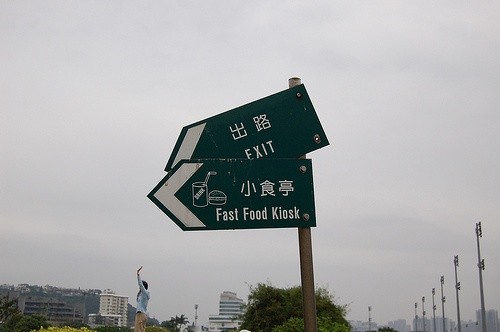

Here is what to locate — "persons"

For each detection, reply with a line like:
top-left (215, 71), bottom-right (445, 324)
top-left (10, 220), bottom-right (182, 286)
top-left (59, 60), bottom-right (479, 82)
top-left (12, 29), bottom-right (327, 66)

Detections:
top-left (134, 266), bottom-right (150, 332)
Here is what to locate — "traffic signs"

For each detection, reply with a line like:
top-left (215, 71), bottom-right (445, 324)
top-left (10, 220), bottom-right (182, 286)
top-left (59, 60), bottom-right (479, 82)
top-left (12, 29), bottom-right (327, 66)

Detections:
top-left (147, 83), bottom-right (331, 231)
